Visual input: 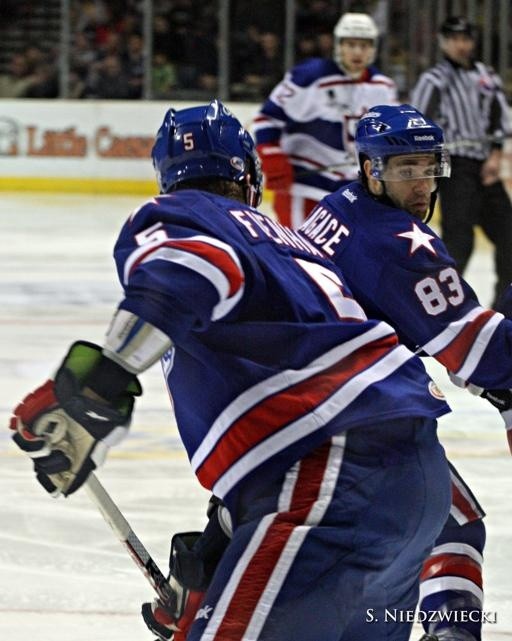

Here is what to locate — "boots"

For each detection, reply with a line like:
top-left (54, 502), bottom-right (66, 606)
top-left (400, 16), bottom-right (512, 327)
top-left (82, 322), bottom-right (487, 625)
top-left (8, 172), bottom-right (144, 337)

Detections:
top-left (142, 602), bottom-right (172, 640)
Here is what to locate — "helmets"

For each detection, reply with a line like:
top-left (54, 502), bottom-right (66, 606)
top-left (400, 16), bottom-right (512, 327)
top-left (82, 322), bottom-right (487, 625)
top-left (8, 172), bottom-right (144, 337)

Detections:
top-left (438, 16), bottom-right (478, 38)
top-left (333, 13), bottom-right (380, 62)
top-left (355, 105), bottom-right (444, 178)
top-left (151, 95), bottom-right (263, 194)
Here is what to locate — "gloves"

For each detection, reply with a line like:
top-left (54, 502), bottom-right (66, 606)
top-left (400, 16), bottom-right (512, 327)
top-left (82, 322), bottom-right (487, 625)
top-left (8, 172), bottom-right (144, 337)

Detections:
top-left (9, 340), bottom-right (143, 499)
top-left (480, 389), bottom-right (512, 454)
top-left (256, 142), bottom-right (293, 191)
top-left (150, 531), bottom-right (203, 632)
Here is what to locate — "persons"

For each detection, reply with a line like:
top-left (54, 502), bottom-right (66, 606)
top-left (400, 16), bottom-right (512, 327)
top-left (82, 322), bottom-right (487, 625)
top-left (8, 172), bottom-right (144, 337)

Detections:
top-left (253, 12), bottom-right (398, 241)
top-left (396, 18), bottom-right (512, 407)
top-left (141, 100), bottom-right (510, 640)
top-left (1, 0), bottom-right (511, 117)
top-left (10, 98), bottom-right (459, 640)
